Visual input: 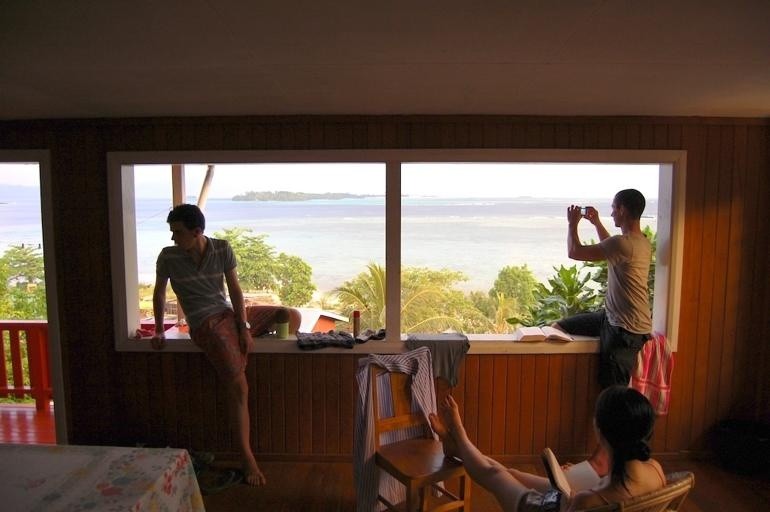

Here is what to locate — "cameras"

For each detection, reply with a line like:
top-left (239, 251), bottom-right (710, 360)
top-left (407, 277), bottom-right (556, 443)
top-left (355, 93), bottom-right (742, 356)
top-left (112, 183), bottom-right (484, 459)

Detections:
top-left (580, 208), bottom-right (587, 216)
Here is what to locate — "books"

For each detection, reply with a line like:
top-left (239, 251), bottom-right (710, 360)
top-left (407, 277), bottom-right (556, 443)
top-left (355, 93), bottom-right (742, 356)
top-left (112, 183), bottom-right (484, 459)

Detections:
top-left (541, 447), bottom-right (600, 494)
top-left (515, 325), bottom-right (571, 342)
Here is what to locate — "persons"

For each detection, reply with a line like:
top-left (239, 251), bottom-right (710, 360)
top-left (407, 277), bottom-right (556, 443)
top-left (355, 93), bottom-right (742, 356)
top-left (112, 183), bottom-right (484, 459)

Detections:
top-left (150, 204), bottom-right (302, 488)
top-left (428, 386), bottom-right (666, 511)
top-left (551, 189), bottom-right (654, 475)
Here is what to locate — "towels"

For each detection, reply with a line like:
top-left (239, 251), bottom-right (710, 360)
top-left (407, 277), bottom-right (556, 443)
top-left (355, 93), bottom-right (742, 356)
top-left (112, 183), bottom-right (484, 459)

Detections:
top-left (626, 333), bottom-right (674, 417)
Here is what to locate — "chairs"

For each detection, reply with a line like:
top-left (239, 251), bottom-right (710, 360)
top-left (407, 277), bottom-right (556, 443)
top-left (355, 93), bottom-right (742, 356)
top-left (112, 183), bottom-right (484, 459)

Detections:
top-left (568, 471), bottom-right (696, 512)
top-left (369, 346), bottom-right (471, 512)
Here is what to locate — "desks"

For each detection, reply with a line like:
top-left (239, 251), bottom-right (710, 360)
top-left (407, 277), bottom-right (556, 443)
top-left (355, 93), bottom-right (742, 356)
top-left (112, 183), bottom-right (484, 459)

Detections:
top-left (0, 443), bottom-right (205, 512)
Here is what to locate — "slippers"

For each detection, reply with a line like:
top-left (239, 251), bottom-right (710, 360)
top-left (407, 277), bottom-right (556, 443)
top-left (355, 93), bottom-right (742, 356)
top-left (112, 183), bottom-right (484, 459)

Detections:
top-left (201, 468), bottom-right (244, 493)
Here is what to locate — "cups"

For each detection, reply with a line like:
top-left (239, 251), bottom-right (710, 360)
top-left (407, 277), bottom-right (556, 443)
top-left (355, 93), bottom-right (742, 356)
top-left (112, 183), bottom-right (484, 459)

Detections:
top-left (275, 323), bottom-right (289, 338)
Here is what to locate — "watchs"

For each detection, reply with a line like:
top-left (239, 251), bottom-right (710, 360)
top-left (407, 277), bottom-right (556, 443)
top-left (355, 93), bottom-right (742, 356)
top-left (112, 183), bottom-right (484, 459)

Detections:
top-left (240, 322), bottom-right (251, 330)
